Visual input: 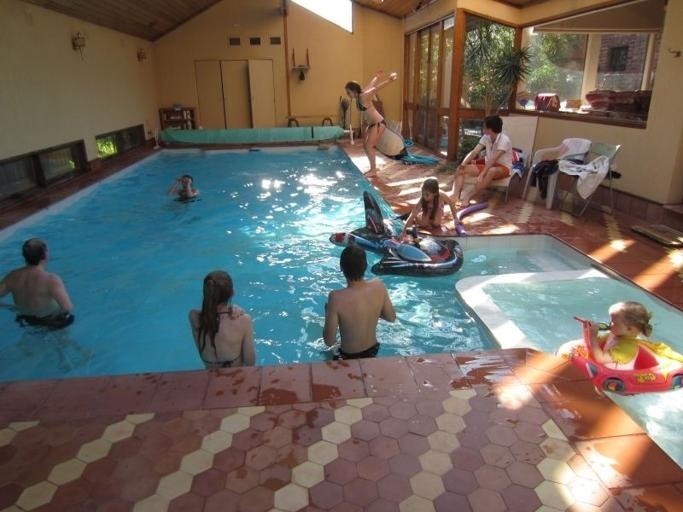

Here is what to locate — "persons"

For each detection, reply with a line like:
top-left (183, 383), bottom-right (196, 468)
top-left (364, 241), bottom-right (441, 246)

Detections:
top-left (0, 238), bottom-right (77, 333)
top-left (397, 179), bottom-right (464, 244)
top-left (449, 115), bottom-right (513, 208)
top-left (168, 174), bottom-right (199, 199)
top-left (582, 300), bottom-right (653, 370)
top-left (322, 245), bottom-right (397, 360)
top-left (345, 70), bottom-right (398, 177)
top-left (190, 270), bottom-right (257, 369)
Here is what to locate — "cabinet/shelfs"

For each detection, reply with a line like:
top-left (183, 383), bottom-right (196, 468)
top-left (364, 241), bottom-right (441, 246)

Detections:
top-left (160, 107), bottom-right (198, 131)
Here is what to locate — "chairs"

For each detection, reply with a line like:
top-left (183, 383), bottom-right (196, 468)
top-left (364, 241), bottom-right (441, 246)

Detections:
top-left (472, 113), bottom-right (540, 199)
top-left (560, 137), bottom-right (621, 219)
top-left (520, 138), bottom-right (593, 211)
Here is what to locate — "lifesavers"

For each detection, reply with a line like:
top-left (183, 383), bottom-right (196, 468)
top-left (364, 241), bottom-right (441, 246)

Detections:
top-left (557, 335), bottom-right (683, 395)
top-left (329, 192), bottom-right (462, 275)
